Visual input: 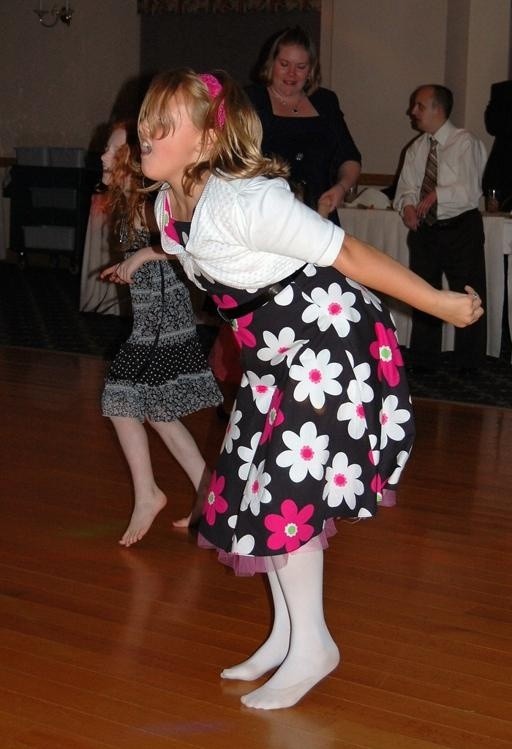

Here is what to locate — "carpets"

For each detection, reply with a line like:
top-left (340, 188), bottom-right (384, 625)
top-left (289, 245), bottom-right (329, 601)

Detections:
top-left (0, 261), bottom-right (512, 409)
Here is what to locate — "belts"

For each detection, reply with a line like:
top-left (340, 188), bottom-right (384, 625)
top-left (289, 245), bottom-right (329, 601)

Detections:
top-left (437, 212), bottom-right (473, 227)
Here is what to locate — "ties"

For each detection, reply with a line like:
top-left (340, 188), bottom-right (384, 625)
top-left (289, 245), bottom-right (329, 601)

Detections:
top-left (421, 138), bottom-right (438, 226)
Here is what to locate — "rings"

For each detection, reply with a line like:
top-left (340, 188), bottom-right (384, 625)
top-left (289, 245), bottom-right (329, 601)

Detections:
top-left (472, 290), bottom-right (479, 302)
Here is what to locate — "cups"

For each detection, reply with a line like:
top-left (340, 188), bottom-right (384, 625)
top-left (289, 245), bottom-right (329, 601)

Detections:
top-left (487, 185), bottom-right (498, 200)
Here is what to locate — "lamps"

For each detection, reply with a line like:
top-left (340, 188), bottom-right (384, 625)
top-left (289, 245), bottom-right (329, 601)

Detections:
top-left (33, 0), bottom-right (76, 30)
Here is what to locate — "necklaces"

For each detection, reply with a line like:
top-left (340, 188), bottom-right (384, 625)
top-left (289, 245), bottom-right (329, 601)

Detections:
top-left (118, 214), bottom-right (128, 244)
top-left (270, 84), bottom-right (307, 114)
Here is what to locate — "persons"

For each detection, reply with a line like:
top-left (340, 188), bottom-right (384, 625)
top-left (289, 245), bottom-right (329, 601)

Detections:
top-left (204, 179), bottom-right (332, 427)
top-left (393, 83), bottom-right (489, 382)
top-left (137, 65), bottom-right (488, 712)
top-left (243, 27), bottom-right (364, 231)
top-left (481, 78), bottom-right (511, 215)
top-left (96, 118), bottom-right (226, 547)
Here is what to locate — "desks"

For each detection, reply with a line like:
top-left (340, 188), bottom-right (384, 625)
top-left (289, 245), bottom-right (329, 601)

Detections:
top-left (338, 200), bottom-right (511, 362)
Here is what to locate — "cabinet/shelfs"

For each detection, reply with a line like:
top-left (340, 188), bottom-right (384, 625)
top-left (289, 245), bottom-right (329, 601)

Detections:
top-left (5, 162), bottom-right (103, 314)
